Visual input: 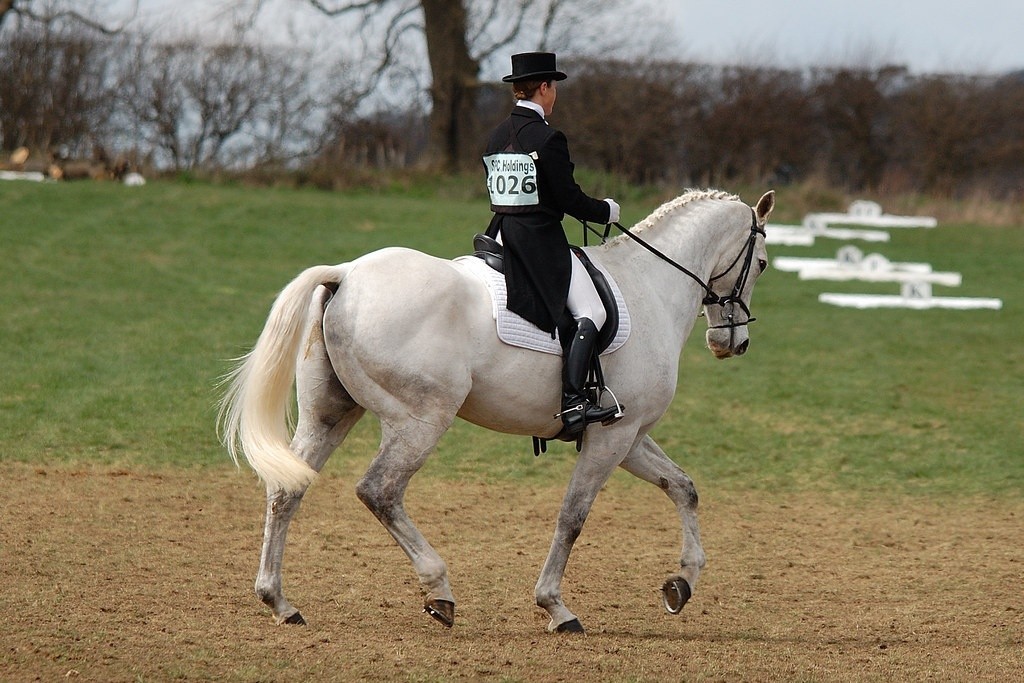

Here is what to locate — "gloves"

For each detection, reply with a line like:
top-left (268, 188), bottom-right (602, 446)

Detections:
top-left (603, 198), bottom-right (621, 223)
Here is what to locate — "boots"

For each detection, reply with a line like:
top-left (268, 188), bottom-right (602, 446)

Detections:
top-left (559, 317), bottom-right (624, 434)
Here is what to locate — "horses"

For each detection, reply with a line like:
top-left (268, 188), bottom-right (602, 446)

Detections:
top-left (209, 186), bottom-right (776, 633)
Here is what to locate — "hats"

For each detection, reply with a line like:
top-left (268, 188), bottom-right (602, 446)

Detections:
top-left (502, 52), bottom-right (568, 83)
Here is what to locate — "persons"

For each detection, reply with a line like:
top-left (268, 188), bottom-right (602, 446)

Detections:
top-left (484, 52), bottom-right (625, 432)
